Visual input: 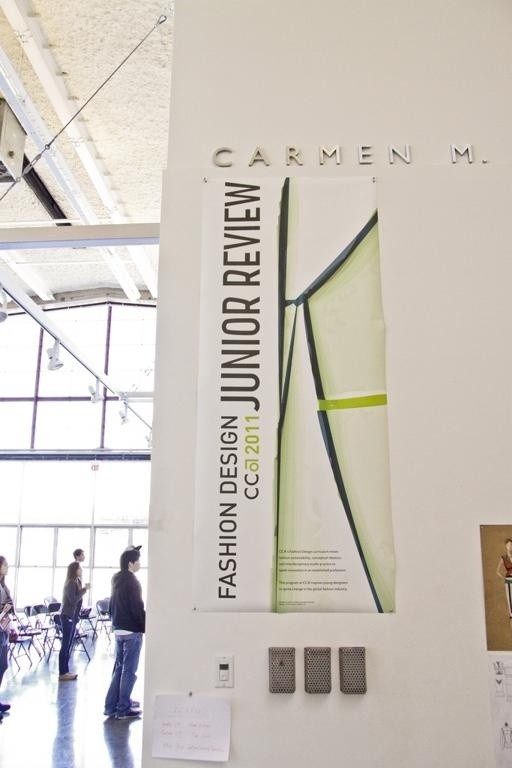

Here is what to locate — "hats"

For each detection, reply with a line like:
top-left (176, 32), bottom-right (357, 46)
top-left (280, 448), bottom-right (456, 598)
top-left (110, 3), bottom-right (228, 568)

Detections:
top-left (124, 544), bottom-right (142, 553)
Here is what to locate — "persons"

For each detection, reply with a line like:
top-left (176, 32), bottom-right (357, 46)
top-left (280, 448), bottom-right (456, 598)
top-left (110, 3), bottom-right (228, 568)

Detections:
top-left (57, 563), bottom-right (92, 680)
top-left (116, 542), bottom-right (142, 710)
top-left (496, 539), bottom-right (512, 621)
top-left (69, 548), bottom-right (86, 651)
top-left (1, 555), bottom-right (17, 723)
top-left (102, 548), bottom-right (147, 718)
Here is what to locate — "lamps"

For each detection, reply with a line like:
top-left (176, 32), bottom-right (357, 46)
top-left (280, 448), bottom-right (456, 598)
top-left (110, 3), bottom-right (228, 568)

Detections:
top-left (0, 295), bottom-right (155, 442)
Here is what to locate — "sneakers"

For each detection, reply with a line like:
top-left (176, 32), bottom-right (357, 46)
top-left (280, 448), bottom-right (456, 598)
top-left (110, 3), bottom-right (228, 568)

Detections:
top-left (58, 672), bottom-right (78, 680)
top-left (0, 702), bottom-right (11, 719)
top-left (104, 698), bottom-right (144, 719)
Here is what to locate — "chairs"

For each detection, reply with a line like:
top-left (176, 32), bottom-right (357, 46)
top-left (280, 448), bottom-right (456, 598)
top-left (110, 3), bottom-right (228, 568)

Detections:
top-left (4, 596), bottom-right (115, 669)
